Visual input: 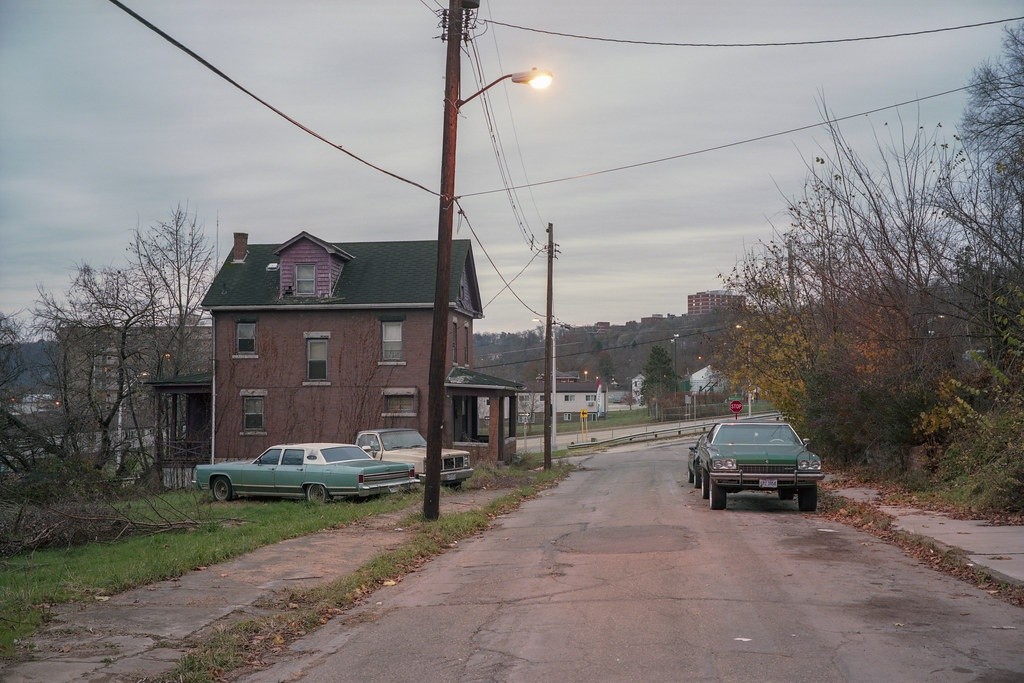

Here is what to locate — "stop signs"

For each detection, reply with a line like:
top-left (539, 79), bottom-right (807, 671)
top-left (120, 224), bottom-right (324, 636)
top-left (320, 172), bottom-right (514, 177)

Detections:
top-left (729, 400), bottom-right (743, 414)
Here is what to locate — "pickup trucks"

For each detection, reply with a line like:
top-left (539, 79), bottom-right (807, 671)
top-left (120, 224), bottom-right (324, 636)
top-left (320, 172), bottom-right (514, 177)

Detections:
top-left (355, 427), bottom-right (475, 491)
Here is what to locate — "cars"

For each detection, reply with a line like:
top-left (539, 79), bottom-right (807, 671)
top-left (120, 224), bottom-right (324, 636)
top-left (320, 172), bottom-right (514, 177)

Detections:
top-left (190, 442), bottom-right (421, 504)
top-left (687, 422), bottom-right (826, 512)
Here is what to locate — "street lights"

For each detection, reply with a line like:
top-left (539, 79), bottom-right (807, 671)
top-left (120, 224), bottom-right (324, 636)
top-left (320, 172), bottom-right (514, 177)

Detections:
top-left (735, 325), bottom-right (752, 417)
top-left (421, 69), bottom-right (557, 520)
